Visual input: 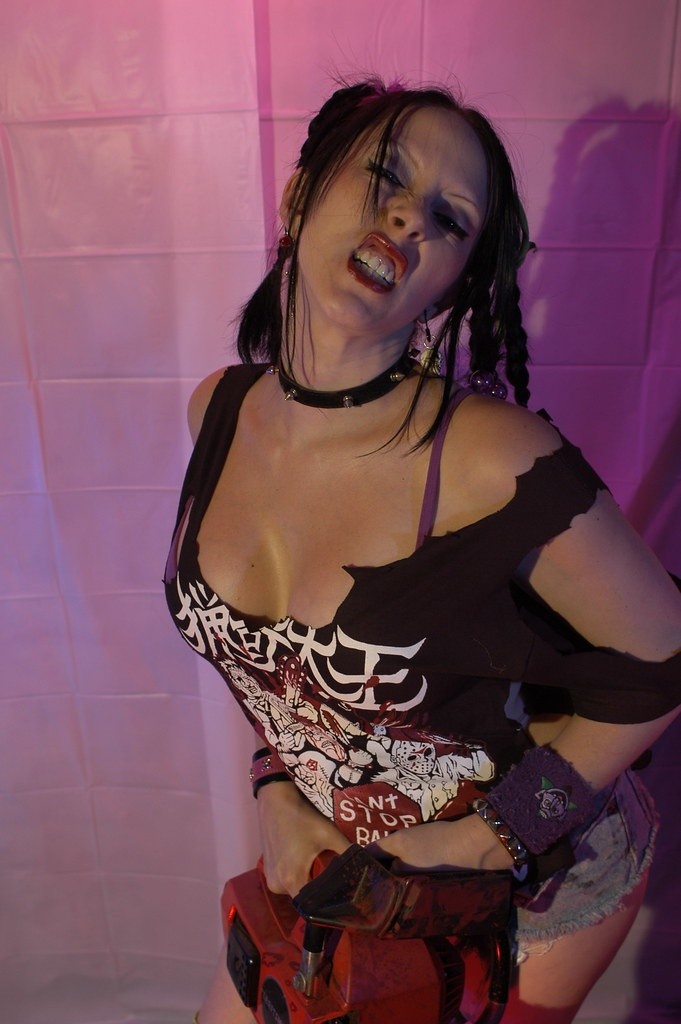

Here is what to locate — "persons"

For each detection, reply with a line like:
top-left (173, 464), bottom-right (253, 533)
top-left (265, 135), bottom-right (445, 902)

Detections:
top-left (160, 77), bottom-right (680, 1024)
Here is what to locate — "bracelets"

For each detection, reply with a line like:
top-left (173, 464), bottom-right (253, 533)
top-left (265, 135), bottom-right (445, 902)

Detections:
top-left (249, 746), bottom-right (296, 801)
top-left (472, 796), bottom-right (530, 867)
top-left (492, 743), bottom-right (601, 859)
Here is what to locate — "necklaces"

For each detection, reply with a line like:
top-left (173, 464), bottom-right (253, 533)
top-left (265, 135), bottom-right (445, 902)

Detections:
top-left (264, 346), bottom-right (422, 409)
